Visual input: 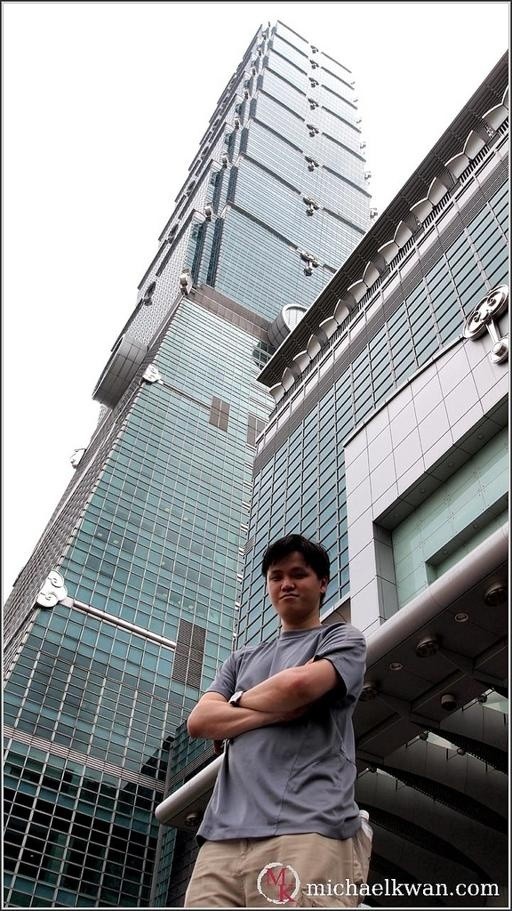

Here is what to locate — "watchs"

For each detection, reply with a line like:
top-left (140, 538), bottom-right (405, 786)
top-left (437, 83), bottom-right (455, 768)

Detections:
top-left (228, 689), bottom-right (248, 708)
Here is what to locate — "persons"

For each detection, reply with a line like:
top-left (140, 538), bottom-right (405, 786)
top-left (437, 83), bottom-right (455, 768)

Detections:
top-left (184, 534), bottom-right (376, 908)
